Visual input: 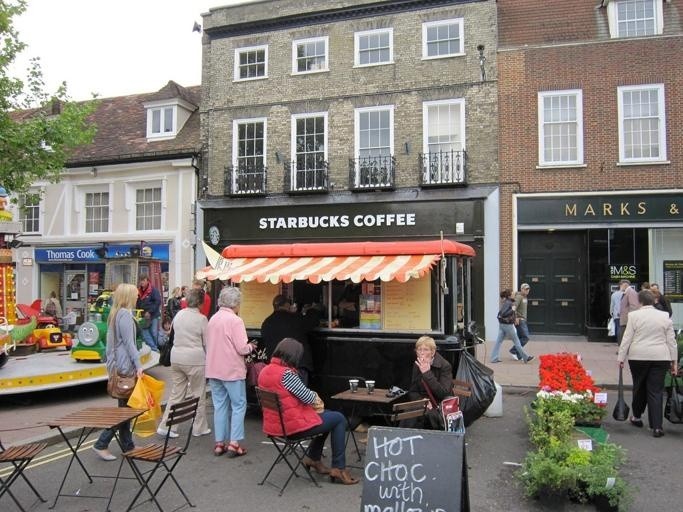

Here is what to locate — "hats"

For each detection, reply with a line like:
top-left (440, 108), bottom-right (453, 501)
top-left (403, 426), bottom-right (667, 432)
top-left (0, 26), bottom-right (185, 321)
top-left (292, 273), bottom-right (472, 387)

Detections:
top-left (520, 283), bottom-right (530, 290)
top-left (618, 279), bottom-right (630, 285)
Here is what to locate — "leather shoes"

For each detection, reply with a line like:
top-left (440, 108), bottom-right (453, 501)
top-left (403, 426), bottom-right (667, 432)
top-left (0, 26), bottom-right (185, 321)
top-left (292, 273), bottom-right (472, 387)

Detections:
top-left (629, 415), bottom-right (642, 427)
top-left (192, 428), bottom-right (211, 437)
top-left (92, 445), bottom-right (117, 461)
top-left (653, 427), bottom-right (664, 437)
top-left (156, 428), bottom-right (179, 438)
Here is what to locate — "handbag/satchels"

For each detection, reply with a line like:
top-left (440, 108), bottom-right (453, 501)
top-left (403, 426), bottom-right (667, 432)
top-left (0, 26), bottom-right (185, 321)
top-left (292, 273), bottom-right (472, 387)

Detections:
top-left (106, 366), bottom-right (137, 400)
top-left (427, 403), bottom-right (444, 430)
top-left (159, 325), bottom-right (174, 367)
top-left (497, 310), bottom-right (515, 324)
top-left (45, 298), bottom-right (55, 315)
top-left (127, 374), bottom-right (164, 438)
top-left (246, 344), bottom-right (267, 387)
top-left (607, 316), bottom-right (615, 336)
top-left (664, 373), bottom-right (682, 424)
top-left (611, 400), bottom-right (629, 421)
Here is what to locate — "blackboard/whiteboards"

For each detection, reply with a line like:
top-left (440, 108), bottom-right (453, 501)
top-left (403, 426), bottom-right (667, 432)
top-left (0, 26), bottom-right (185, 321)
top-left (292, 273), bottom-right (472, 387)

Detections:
top-left (360, 425), bottom-right (470, 512)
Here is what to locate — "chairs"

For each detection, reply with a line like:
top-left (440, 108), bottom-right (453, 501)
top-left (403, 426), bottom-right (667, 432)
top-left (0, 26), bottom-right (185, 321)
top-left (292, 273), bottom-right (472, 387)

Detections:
top-left (0, 439), bottom-right (48, 512)
top-left (119, 396), bottom-right (201, 512)
top-left (447, 379), bottom-right (474, 416)
top-left (257, 387), bottom-right (323, 495)
top-left (389, 399), bottom-right (428, 429)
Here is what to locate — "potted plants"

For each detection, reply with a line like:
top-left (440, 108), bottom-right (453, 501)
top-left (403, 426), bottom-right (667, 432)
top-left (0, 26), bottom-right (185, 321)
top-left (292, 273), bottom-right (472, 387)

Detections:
top-left (516, 400), bottom-right (632, 512)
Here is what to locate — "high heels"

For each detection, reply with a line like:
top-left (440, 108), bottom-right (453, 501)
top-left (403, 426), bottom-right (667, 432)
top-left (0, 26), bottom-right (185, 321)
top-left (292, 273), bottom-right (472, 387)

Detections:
top-left (329, 467), bottom-right (360, 485)
top-left (303, 458), bottom-right (331, 474)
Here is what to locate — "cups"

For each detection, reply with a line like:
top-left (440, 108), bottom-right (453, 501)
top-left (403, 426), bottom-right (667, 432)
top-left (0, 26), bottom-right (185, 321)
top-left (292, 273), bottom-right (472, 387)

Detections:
top-left (348, 379), bottom-right (359, 393)
top-left (365, 380), bottom-right (376, 394)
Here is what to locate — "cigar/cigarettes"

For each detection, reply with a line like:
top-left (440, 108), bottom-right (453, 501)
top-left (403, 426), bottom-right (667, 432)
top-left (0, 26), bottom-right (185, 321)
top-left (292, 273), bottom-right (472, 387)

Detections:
top-left (421, 358), bottom-right (424, 361)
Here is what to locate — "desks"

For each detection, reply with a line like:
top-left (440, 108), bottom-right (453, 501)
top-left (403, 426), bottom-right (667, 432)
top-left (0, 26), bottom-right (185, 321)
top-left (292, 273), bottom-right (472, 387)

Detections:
top-left (330, 386), bottom-right (408, 470)
top-left (43, 404), bottom-right (154, 512)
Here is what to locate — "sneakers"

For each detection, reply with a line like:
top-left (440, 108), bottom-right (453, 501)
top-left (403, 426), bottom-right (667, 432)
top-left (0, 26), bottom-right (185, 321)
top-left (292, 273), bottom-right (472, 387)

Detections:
top-left (508, 352), bottom-right (517, 360)
top-left (525, 356), bottom-right (534, 363)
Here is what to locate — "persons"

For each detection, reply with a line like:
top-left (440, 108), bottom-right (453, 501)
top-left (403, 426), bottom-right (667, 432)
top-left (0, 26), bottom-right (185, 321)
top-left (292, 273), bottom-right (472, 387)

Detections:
top-left (608, 279), bottom-right (671, 357)
top-left (255, 337), bottom-right (361, 485)
top-left (204, 283), bottom-right (258, 460)
top-left (260, 294), bottom-right (315, 387)
top-left (490, 288), bottom-right (536, 363)
top-left (155, 286), bottom-right (212, 438)
top-left (509, 282), bottom-right (530, 360)
top-left (88, 283), bottom-right (140, 461)
top-left (616, 290), bottom-right (680, 437)
top-left (337, 279), bottom-right (360, 327)
top-left (401, 337), bottom-right (453, 428)
top-left (43, 290), bottom-right (62, 319)
top-left (61, 272), bottom-right (213, 353)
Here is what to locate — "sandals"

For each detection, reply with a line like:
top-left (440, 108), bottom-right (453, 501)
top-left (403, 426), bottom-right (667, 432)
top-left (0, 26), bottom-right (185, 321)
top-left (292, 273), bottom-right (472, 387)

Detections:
top-left (227, 443), bottom-right (248, 458)
top-left (214, 444), bottom-right (228, 456)
